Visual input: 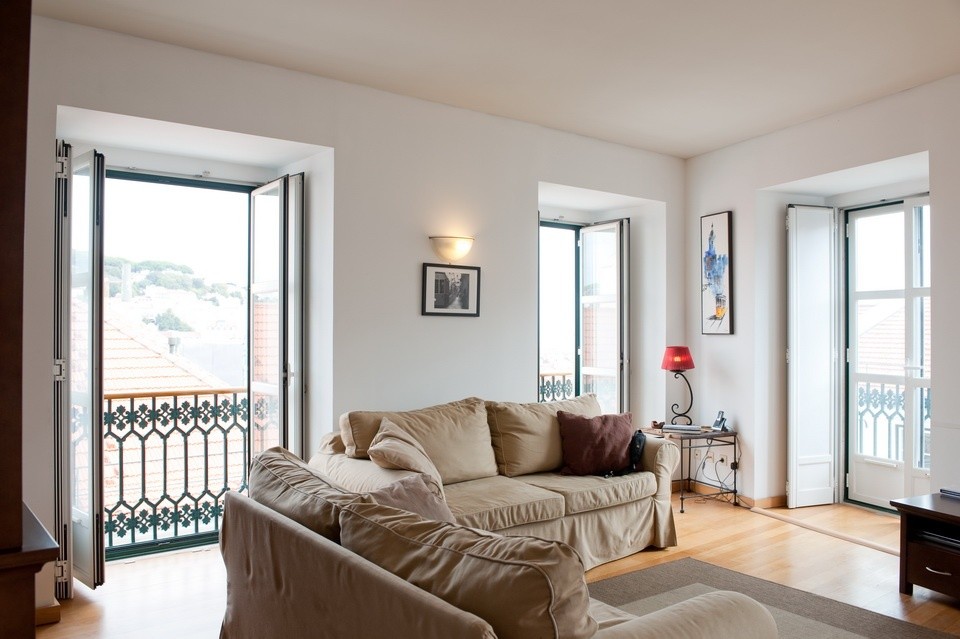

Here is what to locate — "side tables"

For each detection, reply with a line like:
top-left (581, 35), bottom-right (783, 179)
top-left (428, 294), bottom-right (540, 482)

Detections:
top-left (641, 428), bottom-right (740, 514)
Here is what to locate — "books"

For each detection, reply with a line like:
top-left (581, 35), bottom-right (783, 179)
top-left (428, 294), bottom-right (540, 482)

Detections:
top-left (662, 424), bottom-right (701, 435)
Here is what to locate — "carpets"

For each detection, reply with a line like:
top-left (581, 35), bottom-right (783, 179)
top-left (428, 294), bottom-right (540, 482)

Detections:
top-left (586, 557), bottom-right (960, 639)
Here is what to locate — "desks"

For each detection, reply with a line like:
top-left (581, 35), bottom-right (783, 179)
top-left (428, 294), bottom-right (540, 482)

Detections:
top-left (890, 493), bottom-right (960, 597)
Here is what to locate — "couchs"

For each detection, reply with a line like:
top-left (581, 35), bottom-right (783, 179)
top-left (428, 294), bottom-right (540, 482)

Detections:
top-left (308, 393), bottom-right (680, 571)
top-left (220, 446), bottom-right (778, 639)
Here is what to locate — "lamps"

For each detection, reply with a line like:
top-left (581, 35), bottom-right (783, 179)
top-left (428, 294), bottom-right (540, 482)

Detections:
top-left (661, 346), bottom-right (695, 425)
top-left (428, 235), bottom-right (475, 265)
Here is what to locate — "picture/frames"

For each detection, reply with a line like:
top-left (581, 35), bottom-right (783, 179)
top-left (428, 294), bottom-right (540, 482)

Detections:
top-left (421, 263), bottom-right (481, 317)
top-left (700, 211), bottom-right (733, 335)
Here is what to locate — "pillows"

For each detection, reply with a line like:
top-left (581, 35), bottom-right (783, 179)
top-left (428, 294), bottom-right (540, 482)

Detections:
top-left (366, 416), bottom-right (446, 499)
top-left (556, 410), bottom-right (633, 476)
